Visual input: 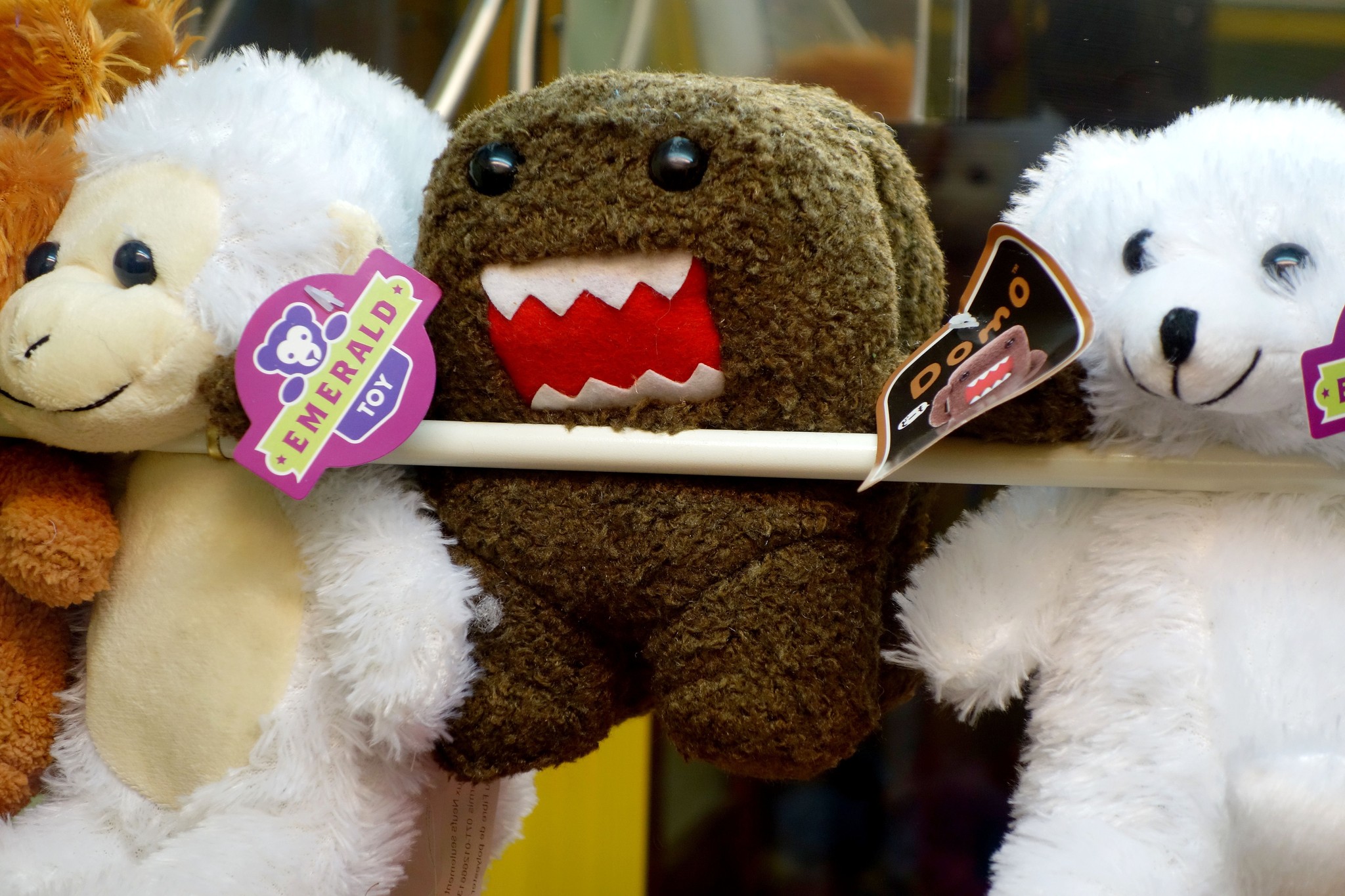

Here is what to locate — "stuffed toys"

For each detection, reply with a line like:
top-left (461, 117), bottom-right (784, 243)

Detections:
top-left (0, 3), bottom-right (220, 823)
top-left (882, 89), bottom-right (1345, 895)
top-left (189, 73), bottom-right (950, 781)
top-left (0, 45), bottom-right (508, 896)
